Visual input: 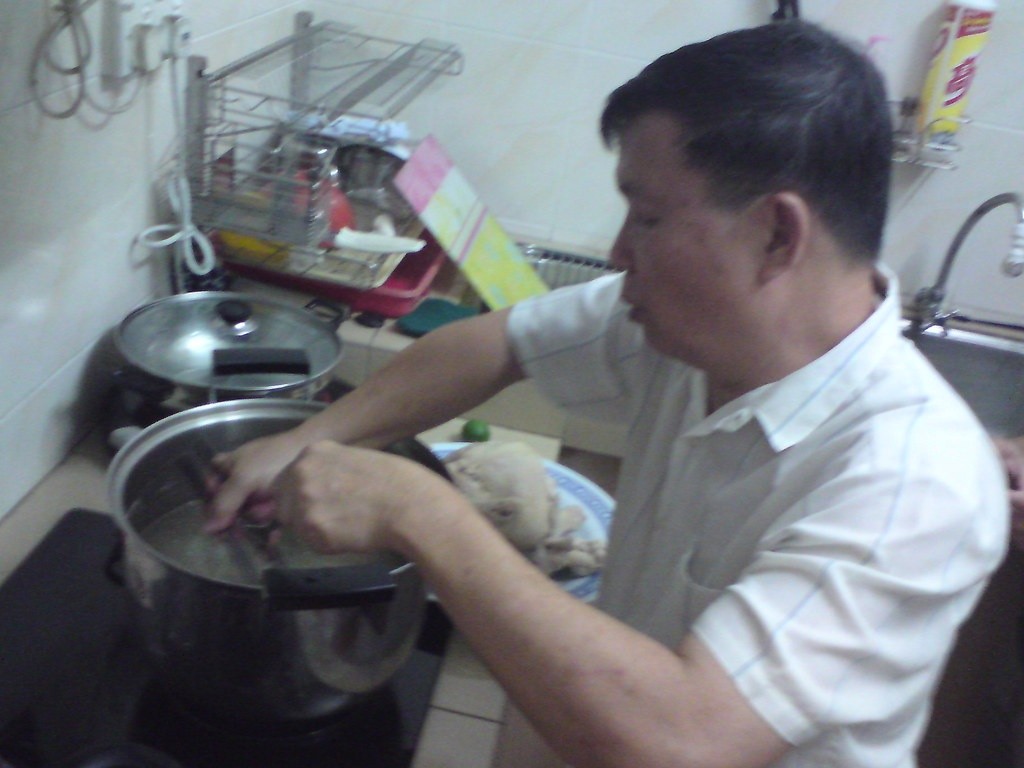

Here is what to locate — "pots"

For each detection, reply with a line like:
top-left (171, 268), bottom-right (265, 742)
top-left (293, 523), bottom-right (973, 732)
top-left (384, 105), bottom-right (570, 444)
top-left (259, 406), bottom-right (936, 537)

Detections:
top-left (105, 348), bottom-right (453, 725)
top-left (109, 290), bottom-right (351, 413)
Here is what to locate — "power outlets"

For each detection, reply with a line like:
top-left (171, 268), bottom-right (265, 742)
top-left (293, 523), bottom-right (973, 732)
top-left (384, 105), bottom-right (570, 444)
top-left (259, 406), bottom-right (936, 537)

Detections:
top-left (130, 3), bottom-right (188, 73)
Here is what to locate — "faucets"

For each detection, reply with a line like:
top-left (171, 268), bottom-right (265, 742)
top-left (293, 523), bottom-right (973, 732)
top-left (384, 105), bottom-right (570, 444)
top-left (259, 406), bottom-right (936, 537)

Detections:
top-left (902, 193), bottom-right (1024, 339)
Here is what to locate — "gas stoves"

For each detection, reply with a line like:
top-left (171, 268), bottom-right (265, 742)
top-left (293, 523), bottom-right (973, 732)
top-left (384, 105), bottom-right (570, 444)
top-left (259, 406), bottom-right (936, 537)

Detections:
top-left (0, 508), bottom-right (452, 768)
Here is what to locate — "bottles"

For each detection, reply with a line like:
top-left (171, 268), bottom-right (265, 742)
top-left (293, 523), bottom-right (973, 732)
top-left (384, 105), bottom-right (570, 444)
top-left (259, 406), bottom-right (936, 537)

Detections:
top-left (915, 0), bottom-right (995, 137)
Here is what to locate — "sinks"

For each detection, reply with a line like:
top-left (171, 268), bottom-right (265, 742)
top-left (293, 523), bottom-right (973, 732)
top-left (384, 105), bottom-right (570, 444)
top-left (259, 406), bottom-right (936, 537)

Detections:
top-left (901, 317), bottom-right (1024, 438)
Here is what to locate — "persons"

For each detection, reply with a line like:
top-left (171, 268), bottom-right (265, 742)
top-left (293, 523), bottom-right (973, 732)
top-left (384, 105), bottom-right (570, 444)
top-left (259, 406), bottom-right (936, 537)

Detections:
top-left (203, 20), bottom-right (1011, 768)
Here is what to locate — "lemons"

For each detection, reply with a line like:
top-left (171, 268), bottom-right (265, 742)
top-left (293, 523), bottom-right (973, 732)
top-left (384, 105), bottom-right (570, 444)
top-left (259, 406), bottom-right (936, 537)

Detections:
top-left (462, 419), bottom-right (489, 442)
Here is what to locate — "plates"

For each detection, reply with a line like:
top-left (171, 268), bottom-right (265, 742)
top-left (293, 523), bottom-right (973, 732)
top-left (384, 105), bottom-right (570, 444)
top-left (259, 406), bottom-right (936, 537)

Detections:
top-left (428, 443), bottom-right (616, 605)
